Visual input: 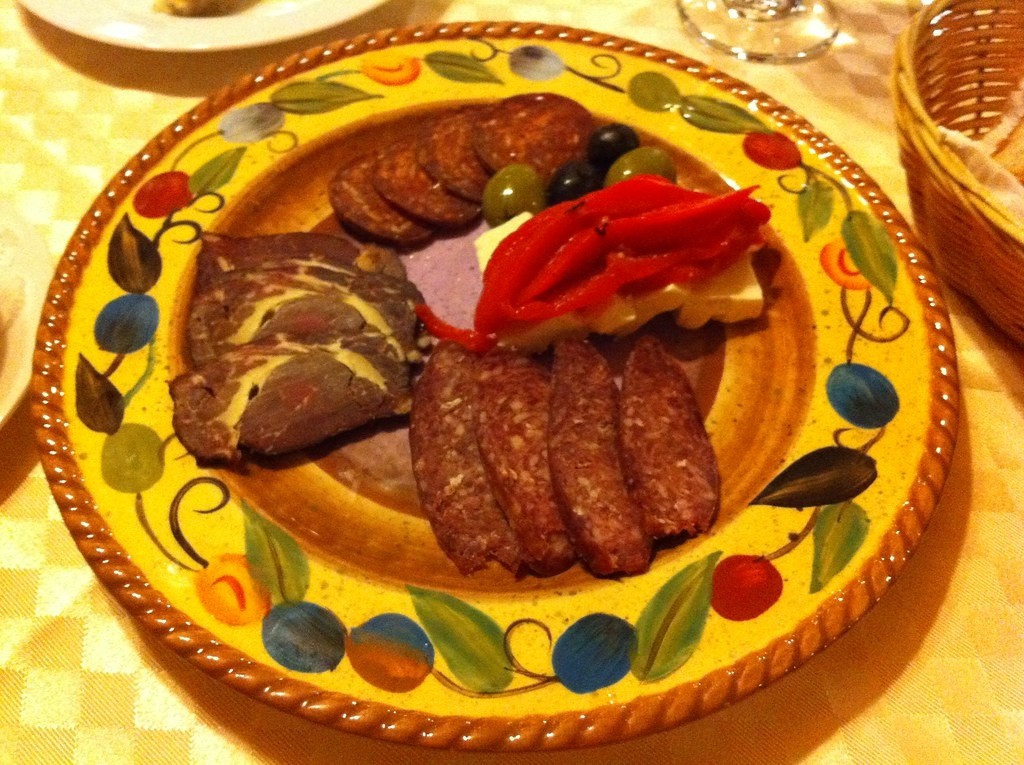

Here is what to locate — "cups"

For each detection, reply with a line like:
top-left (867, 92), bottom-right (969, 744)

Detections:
top-left (676, 0), bottom-right (839, 63)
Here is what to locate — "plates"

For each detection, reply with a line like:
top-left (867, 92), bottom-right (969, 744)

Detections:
top-left (16, 0), bottom-right (390, 53)
top-left (28, 20), bottom-right (961, 752)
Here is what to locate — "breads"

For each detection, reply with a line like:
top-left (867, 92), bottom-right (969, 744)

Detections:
top-left (989, 114), bottom-right (1024, 192)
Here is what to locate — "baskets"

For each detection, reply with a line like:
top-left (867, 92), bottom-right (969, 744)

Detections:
top-left (891, 0), bottom-right (1024, 343)
